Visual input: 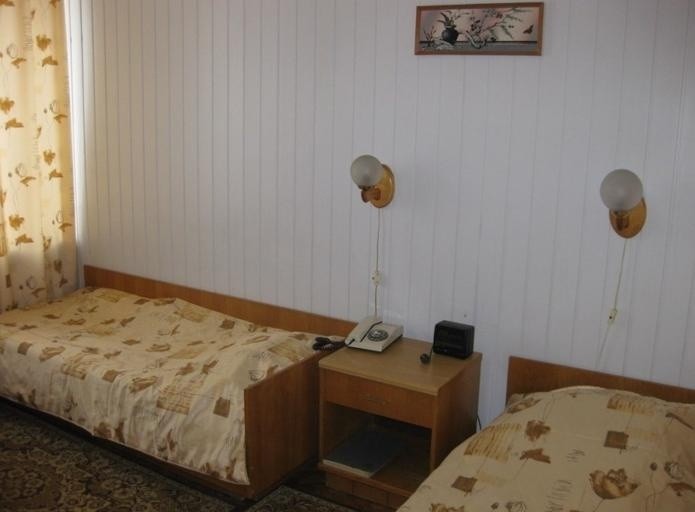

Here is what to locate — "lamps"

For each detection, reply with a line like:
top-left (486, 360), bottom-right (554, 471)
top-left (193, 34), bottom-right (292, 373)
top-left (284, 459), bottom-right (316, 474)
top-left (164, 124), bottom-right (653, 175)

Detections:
top-left (349, 155), bottom-right (395, 208)
top-left (600, 169), bottom-right (647, 238)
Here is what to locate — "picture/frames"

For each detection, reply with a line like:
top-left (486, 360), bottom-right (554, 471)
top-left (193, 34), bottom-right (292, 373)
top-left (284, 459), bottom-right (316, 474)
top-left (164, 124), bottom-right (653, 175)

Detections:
top-left (414, 2), bottom-right (543, 58)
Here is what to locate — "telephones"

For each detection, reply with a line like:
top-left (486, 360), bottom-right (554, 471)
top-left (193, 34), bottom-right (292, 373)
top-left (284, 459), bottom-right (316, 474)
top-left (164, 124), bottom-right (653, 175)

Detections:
top-left (344, 315), bottom-right (404, 353)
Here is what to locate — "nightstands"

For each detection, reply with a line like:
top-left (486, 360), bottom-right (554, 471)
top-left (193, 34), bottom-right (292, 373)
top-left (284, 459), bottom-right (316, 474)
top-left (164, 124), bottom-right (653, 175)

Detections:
top-left (316, 340), bottom-right (482, 509)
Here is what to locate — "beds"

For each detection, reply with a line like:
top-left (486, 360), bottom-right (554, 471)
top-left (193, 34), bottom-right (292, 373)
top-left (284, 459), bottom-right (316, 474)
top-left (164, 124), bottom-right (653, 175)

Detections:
top-left (3, 263), bottom-right (361, 500)
top-left (397, 355), bottom-right (695, 511)
